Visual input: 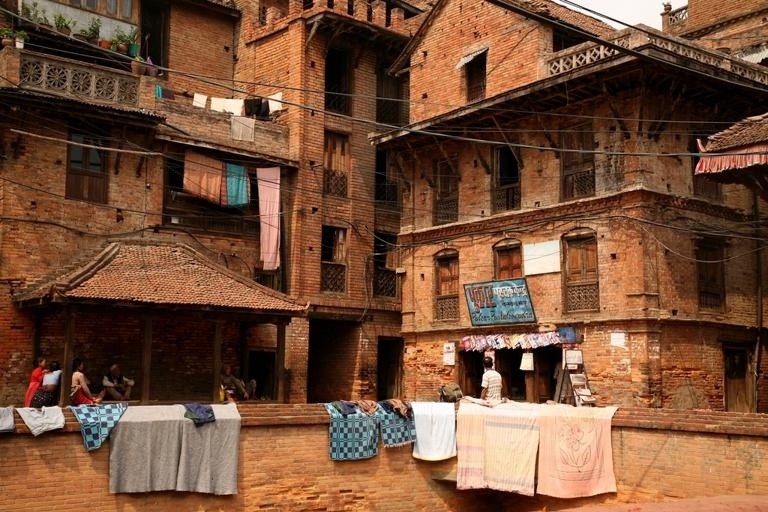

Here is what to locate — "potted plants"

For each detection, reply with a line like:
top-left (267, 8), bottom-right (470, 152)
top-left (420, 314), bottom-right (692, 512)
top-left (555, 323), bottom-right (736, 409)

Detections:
top-left (0, 0), bottom-right (147, 76)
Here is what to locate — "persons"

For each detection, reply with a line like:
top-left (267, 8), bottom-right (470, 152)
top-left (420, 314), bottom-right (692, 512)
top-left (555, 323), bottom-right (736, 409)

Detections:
top-left (480, 357), bottom-right (502, 403)
top-left (221, 364), bottom-right (259, 402)
top-left (25, 357), bottom-right (135, 407)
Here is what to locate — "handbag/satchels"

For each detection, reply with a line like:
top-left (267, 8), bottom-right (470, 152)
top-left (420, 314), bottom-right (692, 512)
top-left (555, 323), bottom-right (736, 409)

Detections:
top-left (519, 350), bottom-right (535, 372)
